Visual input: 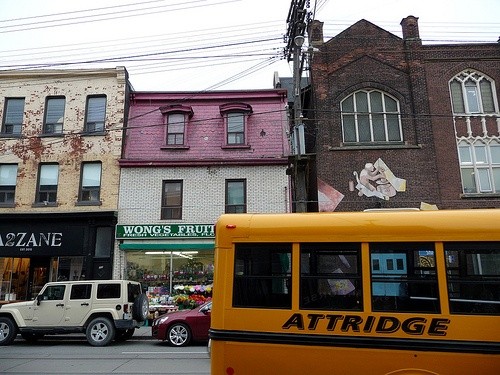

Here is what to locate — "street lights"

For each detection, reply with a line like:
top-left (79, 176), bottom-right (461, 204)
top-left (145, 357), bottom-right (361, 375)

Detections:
top-left (292, 36), bottom-right (310, 214)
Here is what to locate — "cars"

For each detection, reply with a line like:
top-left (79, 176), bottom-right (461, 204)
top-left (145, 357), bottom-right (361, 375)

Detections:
top-left (154, 298), bottom-right (212, 347)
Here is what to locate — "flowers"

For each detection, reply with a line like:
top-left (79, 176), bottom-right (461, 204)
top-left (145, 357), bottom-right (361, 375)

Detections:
top-left (172, 284), bottom-right (212, 308)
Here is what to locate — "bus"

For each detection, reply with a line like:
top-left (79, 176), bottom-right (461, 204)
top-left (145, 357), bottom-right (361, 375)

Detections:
top-left (210, 209), bottom-right (500, 375)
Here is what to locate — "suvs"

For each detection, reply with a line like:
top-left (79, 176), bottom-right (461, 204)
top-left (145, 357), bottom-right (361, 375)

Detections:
top-left (0, 280), bottom-right (150, 347)
